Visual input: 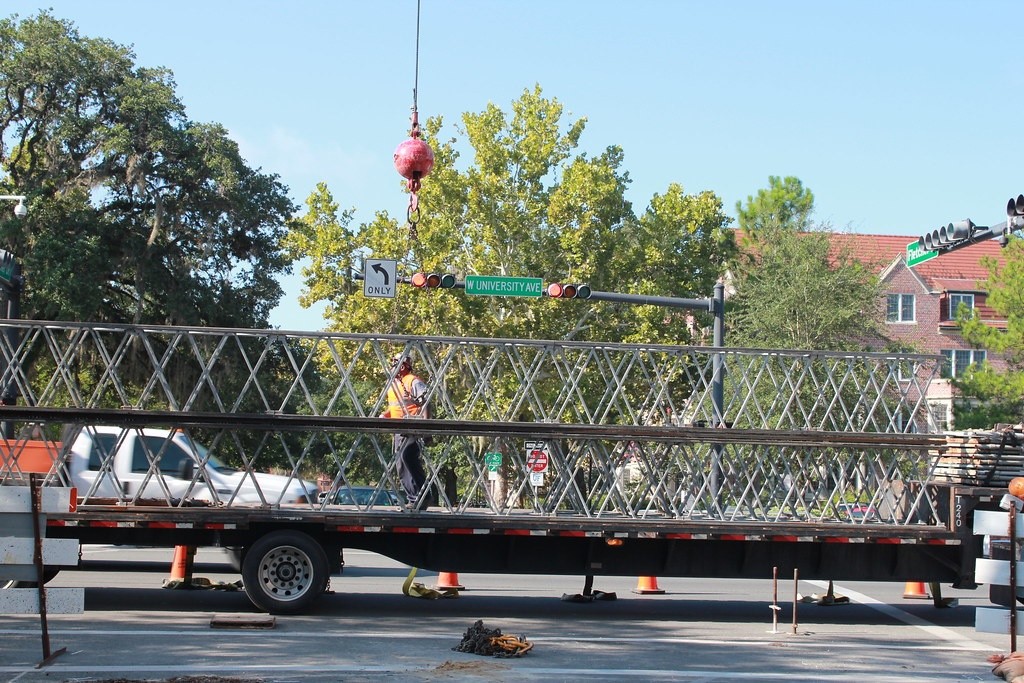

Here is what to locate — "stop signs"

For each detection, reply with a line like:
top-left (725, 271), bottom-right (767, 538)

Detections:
top-left (526, 449), bottom-right (548, 473)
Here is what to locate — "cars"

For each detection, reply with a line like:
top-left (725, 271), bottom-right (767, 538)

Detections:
top-left (318, 483), bottom-right (410, 507)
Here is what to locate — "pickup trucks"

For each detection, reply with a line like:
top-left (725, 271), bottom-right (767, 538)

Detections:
top-left (0, 423), bottom-right (322, 508)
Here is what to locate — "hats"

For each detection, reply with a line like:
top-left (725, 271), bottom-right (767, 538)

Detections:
top-left (389, 352), bottom-right (413, 367)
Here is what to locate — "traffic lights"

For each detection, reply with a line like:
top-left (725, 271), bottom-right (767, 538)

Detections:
top-left (918, 219), bottom-right (976, 252)
top-left (547, 282), bottom-right (592, 299)
top-left (1006, 194), bottom-right (1024, 217)
top-left (410, 272), bottom-right (457, 291)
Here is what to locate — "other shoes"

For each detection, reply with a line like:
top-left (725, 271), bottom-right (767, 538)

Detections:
top-left (400, 505), bottom-right (427, 513)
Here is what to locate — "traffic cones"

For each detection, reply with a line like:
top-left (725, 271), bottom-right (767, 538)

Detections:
top-left (432, 571), bottom-right (465, 590)
top-left (901, 581), bottom-right (929, 599)
top-left (631, 576), bottom-right (665, 595)
top-left (165, 545), bottom-right (185, 586)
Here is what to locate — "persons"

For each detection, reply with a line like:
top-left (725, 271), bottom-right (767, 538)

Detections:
top-left (378, 350), bottom-right (435, 512)
